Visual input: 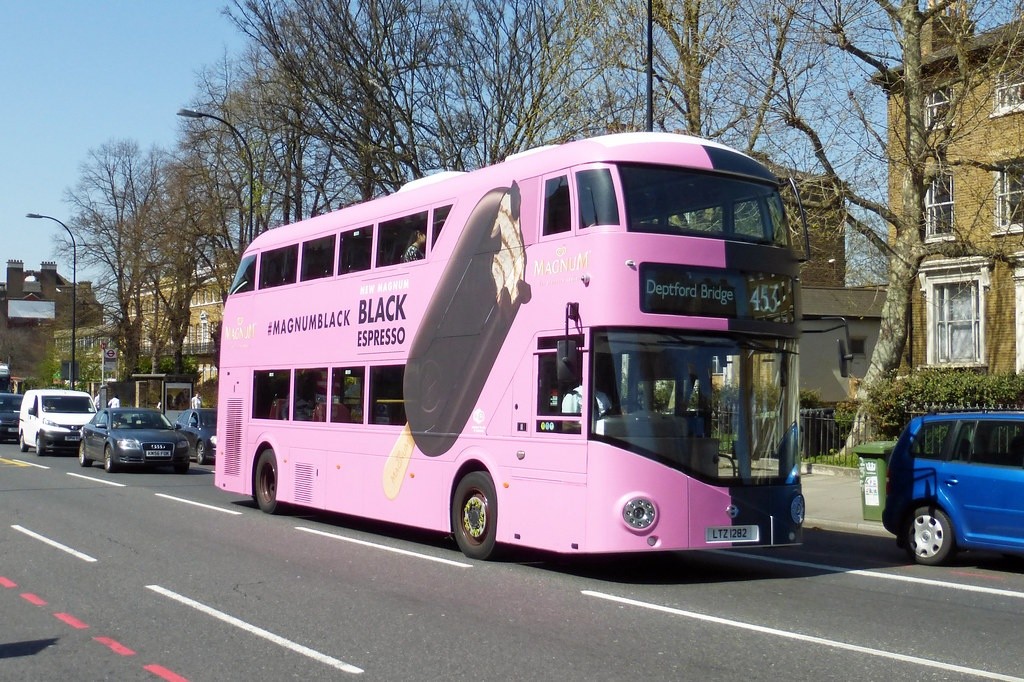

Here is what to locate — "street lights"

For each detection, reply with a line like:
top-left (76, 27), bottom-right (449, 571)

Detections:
top-left (176, 108), bottom-right (253, 244)
top-left (25, 212), bottom-right (76, 390)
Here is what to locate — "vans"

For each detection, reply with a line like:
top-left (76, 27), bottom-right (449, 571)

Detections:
top-left (18, 388), bottom-right (100, 457)
top-left (882, 408), bottom-right (1024, 566)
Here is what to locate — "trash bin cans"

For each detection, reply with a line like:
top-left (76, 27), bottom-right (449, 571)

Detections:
top-left (851, 440), bottom-right (895, 521)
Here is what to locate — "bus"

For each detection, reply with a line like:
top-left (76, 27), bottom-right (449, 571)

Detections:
top-left (214, 131), bottom-right (855, 562)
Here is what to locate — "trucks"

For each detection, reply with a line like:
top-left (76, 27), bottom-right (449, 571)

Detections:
top-left (0, 364), bottom-right (11, 393)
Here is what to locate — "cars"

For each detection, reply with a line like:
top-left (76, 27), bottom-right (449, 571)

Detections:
top-left (79, 407), bottom-right (191, 474)
top-left (172, 408), bottom-right (217, 466)
top-left (0, 393), bottom-right (24, 444)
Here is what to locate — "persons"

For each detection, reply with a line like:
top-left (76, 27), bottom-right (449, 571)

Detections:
top-left (108, 394), bottom-right (121, 418)
top-left (157, 389), bottom-right (189, 409)
top-left (400, 228), bottom-right (426, 263)
top-left (191, 391), bottom-right (203, 410)
top-left (561, 385), bottom-right (613, 429)
top-left (94, 391), bottom-right (102, 410)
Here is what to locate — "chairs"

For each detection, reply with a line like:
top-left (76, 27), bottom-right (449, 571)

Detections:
top-left (960, 439), bottom-right (970, 459)
top-left (1008, 435), bottom-right (1024, 463)
